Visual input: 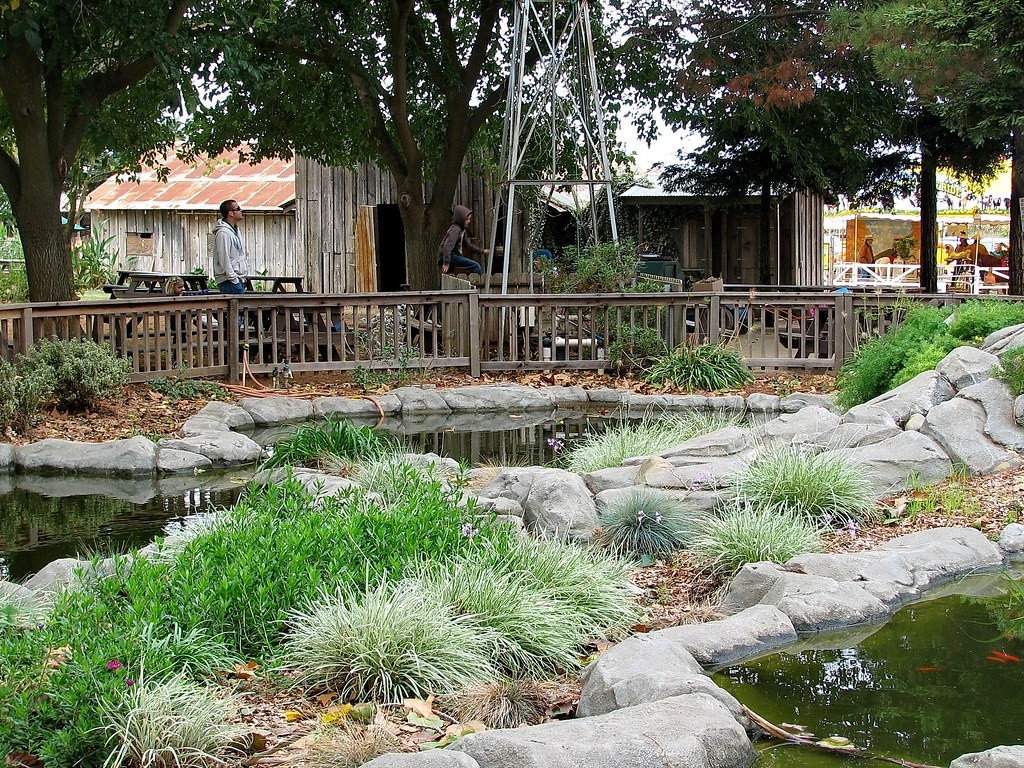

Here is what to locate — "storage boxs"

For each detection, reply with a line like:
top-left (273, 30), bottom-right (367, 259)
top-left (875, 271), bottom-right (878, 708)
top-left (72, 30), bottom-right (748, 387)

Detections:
top-left (692, 278), bottom-right (724, 291)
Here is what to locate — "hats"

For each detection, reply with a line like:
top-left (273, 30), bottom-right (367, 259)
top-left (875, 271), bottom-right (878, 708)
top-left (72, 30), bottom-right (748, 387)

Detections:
top-left (972, 234), bottom-right (981, 240)
top-left (864, 234), bottom-right (874, 240)
top-left (959, 230), bottom-right (968, 239)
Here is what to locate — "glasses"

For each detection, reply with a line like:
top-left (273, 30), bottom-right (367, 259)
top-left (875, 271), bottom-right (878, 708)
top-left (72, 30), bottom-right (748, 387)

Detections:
top-left (231, 206), bottom-right (240, 211)
top-left (894, 238), bottom-right (903, 241)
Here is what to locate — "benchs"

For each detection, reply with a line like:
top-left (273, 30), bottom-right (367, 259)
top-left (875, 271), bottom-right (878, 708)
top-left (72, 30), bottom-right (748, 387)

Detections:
top-left (778, 332), bottom-right (814, 352)
top-left (92, 270), bottom-right (342, 372)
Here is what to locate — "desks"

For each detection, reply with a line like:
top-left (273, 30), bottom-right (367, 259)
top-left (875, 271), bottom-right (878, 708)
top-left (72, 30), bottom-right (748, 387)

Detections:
top-left (128, 273), bottom-right (210, 292)
top-left (788, 307), bottom-right (897, 375)
top-left (109, 269), bottom-right (152, 298)
top-left (244, 275), bottom-right (305, 294)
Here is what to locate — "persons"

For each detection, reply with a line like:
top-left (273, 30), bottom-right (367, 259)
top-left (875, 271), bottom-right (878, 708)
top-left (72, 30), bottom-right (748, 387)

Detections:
top-left (165, 276), bottom-right (187, 361)
top-left (857, 234), bottom-right (875, 278)
top-left (954, 235), bottom-right (969, 270)
top-left (212, 200), bottom-right (249, 328)
top-left (965, 233), bottom-right (989, 270)
top-left (427, 205), bottom-right (490, 328)
top-left (993, 243), bottom-right (1002, 251)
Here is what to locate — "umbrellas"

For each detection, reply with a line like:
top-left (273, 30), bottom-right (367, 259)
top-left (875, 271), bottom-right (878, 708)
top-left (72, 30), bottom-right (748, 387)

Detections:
top-left (61, 216), bottom-right (85, 232)
